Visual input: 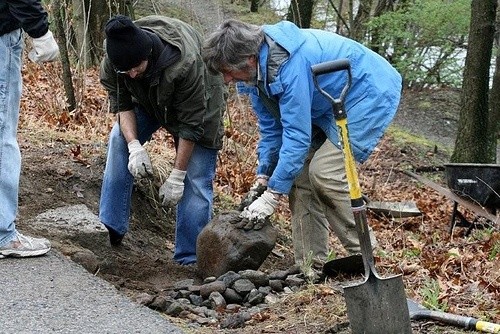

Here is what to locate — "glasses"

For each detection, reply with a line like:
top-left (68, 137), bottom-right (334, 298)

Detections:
top-left (114, 59), bottom-right (144, 73)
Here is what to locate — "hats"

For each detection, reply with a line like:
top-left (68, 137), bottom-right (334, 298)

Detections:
top-left (104, 14), bottom-right (153, 69)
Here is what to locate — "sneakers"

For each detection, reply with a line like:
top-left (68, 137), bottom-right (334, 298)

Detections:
top-left (0, 233), bottom-right (51, 259)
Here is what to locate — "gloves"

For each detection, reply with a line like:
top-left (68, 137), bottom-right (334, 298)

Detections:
top-left (158, 168), bottom-right (187, 207)
top-left (28, 30), bottom-right (59, 63)
top-left (228, 191), bottom-right (279, 230)
top-left (238, 181), bottom-right (268, 211)
top-left (128, 140), bottom-right (153, 179)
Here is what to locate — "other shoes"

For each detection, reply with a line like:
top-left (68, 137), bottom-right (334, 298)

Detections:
top-left (101, 222), bottom-right (124, 247)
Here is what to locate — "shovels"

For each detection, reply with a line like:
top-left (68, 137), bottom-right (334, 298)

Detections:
top-left (311, 58), bottom-right (413, 334)
top-left (406, 298), bottom-right (500, 334)
top-left (361, 193), bottom-right (421, 216)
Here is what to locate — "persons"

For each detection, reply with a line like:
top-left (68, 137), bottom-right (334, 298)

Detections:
top-left (203, 17), bottom-right (404, 269)
top-left (100, 15), bottom-right (228, 265)
top-left (0, 0), bottom-right (59, 259)
